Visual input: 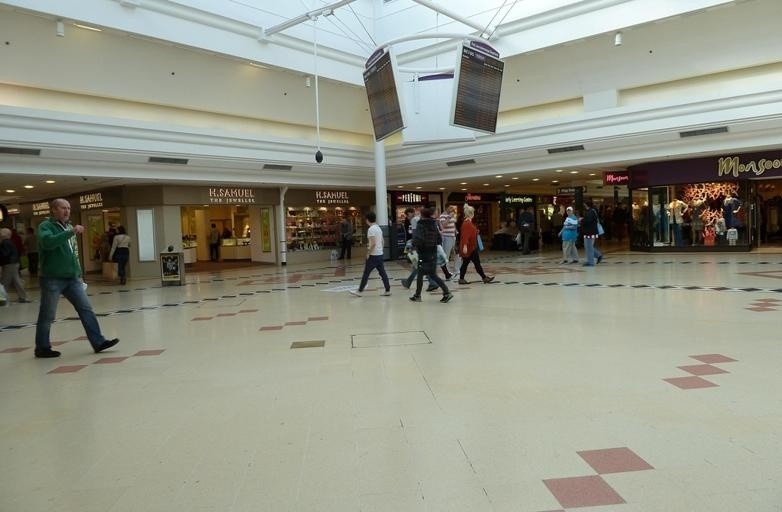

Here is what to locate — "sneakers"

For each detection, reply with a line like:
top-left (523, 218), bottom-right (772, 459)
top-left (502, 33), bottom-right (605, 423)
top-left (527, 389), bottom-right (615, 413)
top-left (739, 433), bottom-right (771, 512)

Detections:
top-left (35, 346), bottom-right (60, 357)
top-left (95, 339), bottom-right (119, 354)
top-left (349, 273), bottom-right (495, 303)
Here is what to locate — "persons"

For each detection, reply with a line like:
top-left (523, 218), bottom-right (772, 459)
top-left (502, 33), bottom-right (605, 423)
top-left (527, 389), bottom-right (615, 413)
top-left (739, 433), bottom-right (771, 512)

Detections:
top-left (632, 195), bottom-right (742, 246)
top-left (579, 201), bottom-right (603, 266)
top-left (548, 202), bottom-right (630, 241)
top-left (336, 219), bottom-right (352, 261)
top-left (518, 207), bottom-right (533, 250)
top-left (458, 203), bottom-right (494, 284)
top-left (0, 218), bottom-right (132, 306)
top-left (207, 223), bottom-right (222, 263)
top-left (162, 256), bottom-right (178, 276)
top-left (35, 198), bottom-right (119, 359)
top-left (221, 225), bottom-right (231, 240)
top-left (402, 204), bottom-right (460, 304)
top-left (557, 206), bottom-right (580, 264)
top-left (349, 211), bottom-right (393, 297)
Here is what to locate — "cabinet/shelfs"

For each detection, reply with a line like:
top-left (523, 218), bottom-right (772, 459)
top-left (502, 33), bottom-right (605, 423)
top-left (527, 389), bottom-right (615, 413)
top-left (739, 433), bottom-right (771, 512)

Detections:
top-left (221, 238), bottom-right (251, 259)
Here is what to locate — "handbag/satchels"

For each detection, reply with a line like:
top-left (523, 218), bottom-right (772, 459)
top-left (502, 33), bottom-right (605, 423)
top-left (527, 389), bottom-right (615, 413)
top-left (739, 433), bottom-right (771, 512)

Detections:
top-left (102, 262), bottom-right (118, 282)
top-left (596, 221), bottom-right (604, 235)
top-left (477, 234), bottom-right (484, 251)
